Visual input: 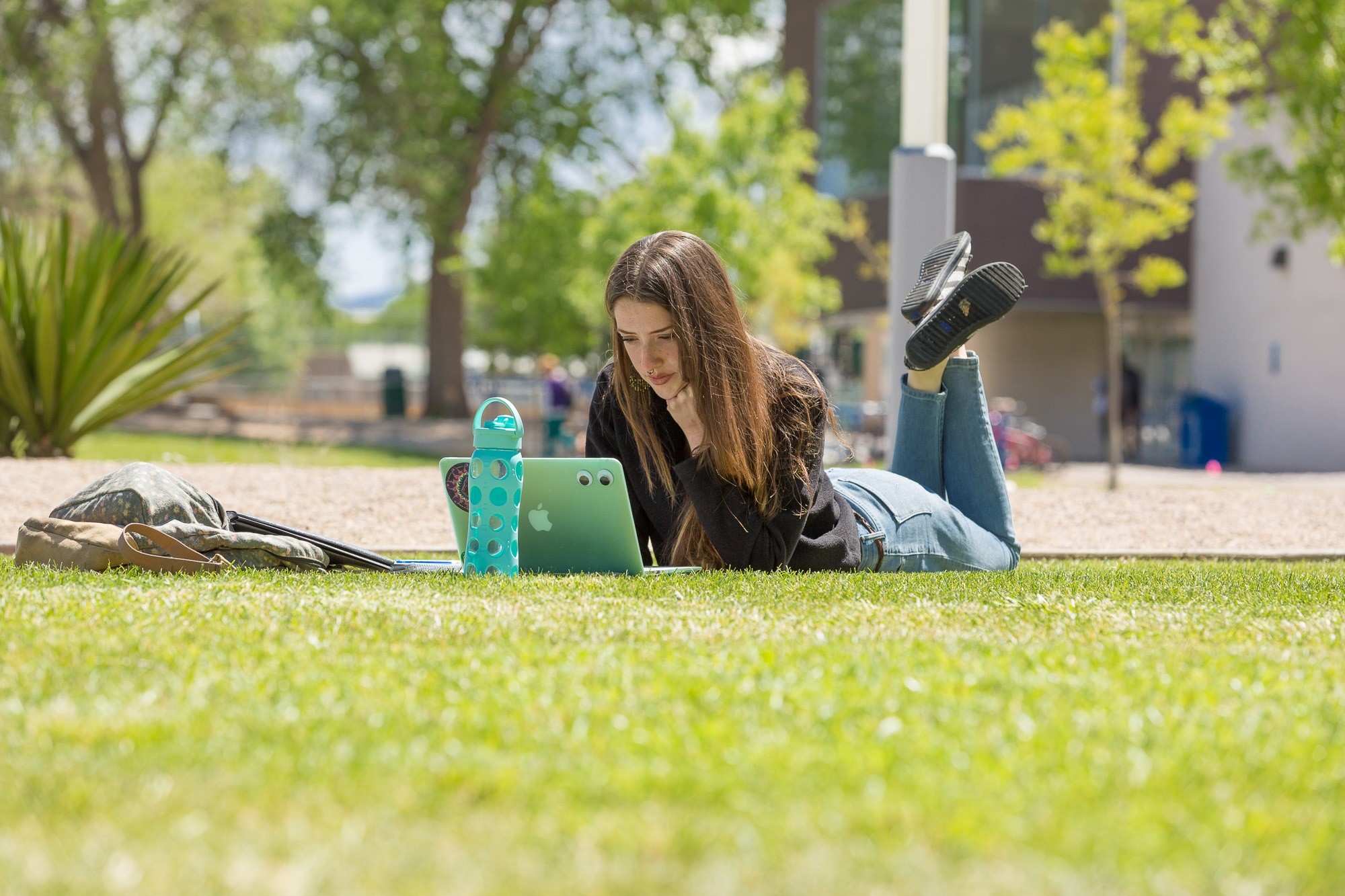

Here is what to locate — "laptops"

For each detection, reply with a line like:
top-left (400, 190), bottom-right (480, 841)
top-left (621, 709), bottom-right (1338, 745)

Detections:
top-left (439, 457), bottom-right (704, 576)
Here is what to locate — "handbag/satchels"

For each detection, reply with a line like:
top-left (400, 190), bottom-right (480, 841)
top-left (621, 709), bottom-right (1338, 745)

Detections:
top-left (16, 463), bottom-right (330, 570)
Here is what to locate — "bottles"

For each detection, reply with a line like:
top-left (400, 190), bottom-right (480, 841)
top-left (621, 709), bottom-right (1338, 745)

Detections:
top-left (463, 397), bottom-right (523, 578)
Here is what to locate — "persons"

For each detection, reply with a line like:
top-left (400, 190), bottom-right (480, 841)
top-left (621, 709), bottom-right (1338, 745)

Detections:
top-left (585, 230), bottom-right (1025, 572)
top-left (1121, 360), bottom-right (1140, 464)
top-left (536, 354), bottom-right (576, 458)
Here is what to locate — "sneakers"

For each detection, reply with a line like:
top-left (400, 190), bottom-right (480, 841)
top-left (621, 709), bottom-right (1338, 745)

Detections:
top-left (902, 262), bottom-right (1026, 371)
top-left (902, 230), bottom-right (973, 325)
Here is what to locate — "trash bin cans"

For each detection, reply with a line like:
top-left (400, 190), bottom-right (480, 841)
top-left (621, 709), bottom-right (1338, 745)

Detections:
top-left (382, 370), bottom-right (408, 413)
top-left (1181, 390), bottom-right (1229, 466)
